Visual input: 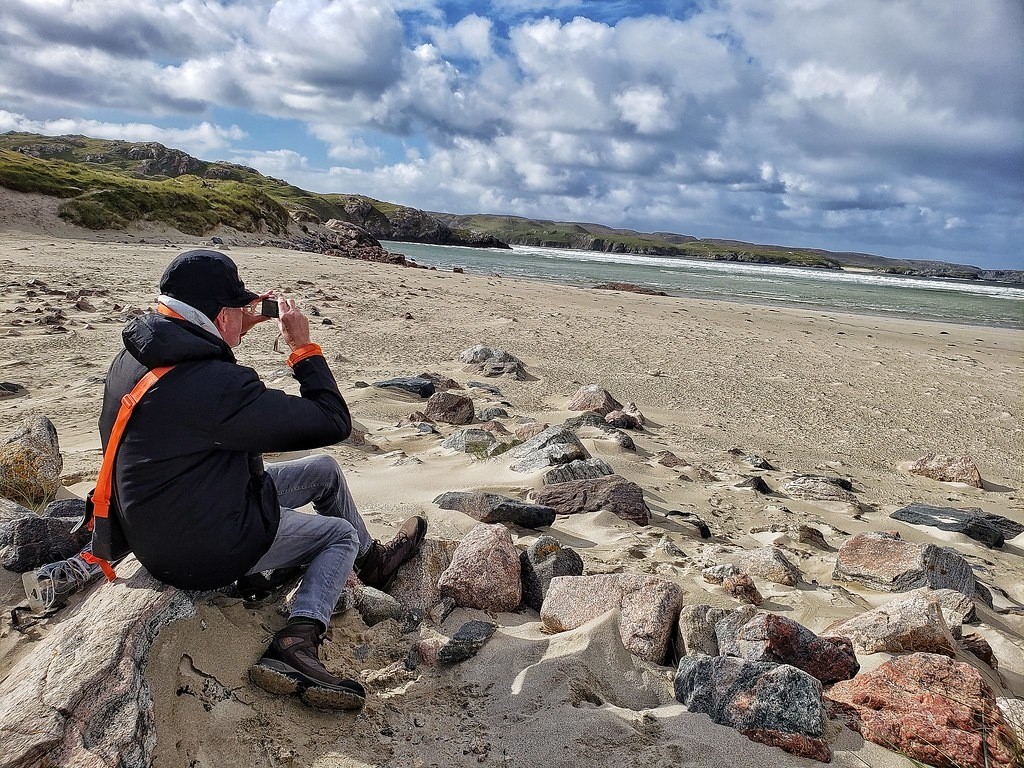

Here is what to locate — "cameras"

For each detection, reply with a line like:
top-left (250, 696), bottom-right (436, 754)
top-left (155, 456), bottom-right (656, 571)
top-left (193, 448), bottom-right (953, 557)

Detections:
top-left (261, 298), bottom-right (279, 318)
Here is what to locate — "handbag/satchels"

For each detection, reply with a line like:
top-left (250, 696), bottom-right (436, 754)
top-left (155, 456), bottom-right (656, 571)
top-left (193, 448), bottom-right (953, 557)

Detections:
top-left (70, 484), bottom-right (132, 562)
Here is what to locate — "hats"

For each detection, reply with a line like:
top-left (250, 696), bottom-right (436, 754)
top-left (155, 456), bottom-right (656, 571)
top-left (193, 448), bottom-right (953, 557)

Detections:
top-left (159, 249), bottom-right (260, 307)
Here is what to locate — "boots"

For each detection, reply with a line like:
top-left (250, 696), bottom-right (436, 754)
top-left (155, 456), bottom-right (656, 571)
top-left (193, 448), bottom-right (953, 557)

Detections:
top-left (250, 621), bottom-right (366, 711)
top-left (354, 515), bottom-right (427, 593)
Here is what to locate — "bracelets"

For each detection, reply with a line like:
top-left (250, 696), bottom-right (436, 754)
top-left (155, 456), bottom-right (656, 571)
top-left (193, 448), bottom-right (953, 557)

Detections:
top-left (287, 343), bottom-right (323, 370)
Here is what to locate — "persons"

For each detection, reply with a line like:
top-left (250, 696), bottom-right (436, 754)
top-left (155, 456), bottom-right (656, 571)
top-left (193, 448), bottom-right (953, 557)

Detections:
top-left (97, 249), bottom-right (427, 713)
